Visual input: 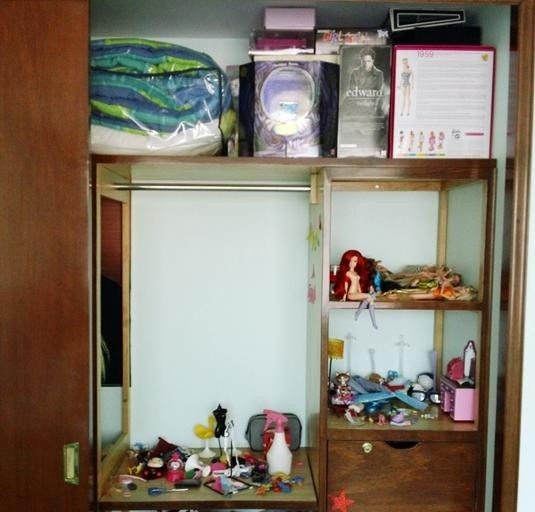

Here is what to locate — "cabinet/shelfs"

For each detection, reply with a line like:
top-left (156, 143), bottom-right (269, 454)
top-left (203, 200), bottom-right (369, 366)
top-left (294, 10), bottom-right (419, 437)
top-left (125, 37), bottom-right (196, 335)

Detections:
top-left (307, 166), bottom-right (497, 512)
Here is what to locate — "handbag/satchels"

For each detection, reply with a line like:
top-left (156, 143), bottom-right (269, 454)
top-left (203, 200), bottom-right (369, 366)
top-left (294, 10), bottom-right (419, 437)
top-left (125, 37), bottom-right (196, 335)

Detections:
top-left (245, 413), bottom-right (302, 450)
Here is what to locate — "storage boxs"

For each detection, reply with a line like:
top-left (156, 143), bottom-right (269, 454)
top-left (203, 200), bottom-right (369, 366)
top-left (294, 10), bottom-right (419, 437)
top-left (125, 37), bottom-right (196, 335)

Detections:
top-left (389, 44), bottom-right (497, 160)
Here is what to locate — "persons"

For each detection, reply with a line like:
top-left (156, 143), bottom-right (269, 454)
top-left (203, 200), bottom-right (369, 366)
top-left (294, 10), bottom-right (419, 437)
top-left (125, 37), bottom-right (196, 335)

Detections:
top-left (326, 247), bottom-right (479, 426)
top-left (397, 57), bottom-right (415, 119)
top-left (349, 46), bottom-right (386, 112)
